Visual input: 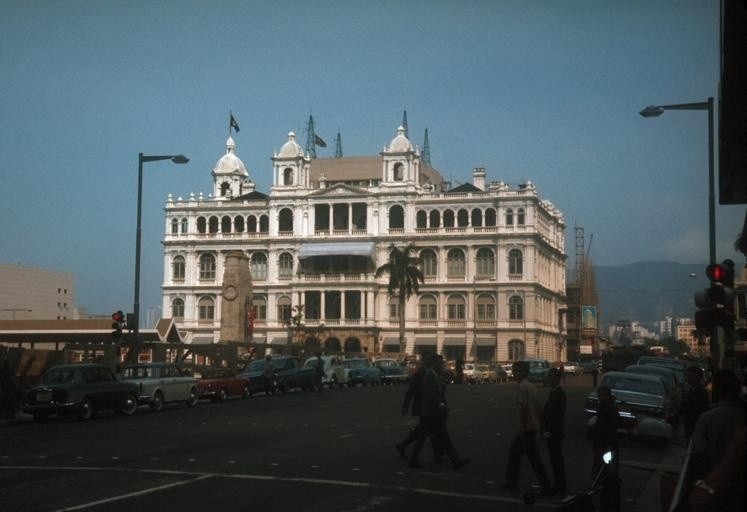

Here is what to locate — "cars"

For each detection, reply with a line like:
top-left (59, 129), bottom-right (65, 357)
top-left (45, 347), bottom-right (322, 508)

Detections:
top-left (583, 370), bottom-right (679, 437)
top-left (624, 356), bottom-right (709, 412)
top-left (462, 363), bottom-right (483, 385)
top-left (342, 358), bottom-right (381, 386)
top-left (374, 357), bottom-right (603, 385)
top-left (196, 367), bottom-right (251, 400)
top-left (303, 355), bottom-right (354, 389)
top-left (239, 355), bottom-right (318, 394)
top-left (479, 365), bottom-right (499, 383)
top-left (20, 363), bottom-right (139, 422)
top-left (118, 361), bottom-right (201, 411)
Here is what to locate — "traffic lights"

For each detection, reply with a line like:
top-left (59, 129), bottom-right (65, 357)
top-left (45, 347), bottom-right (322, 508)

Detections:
top-left (111, 311), bottom-right (124, 342)
top-left (694, 286), bottom-right (715, 334)
top-left (705, 254), bottom-right (734, 329)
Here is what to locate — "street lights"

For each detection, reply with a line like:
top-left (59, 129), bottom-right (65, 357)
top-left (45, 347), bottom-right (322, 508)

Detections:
top-left (133, 152), bottom-right (188, 338)
top-left (637, 96), bottom-right (718, 262)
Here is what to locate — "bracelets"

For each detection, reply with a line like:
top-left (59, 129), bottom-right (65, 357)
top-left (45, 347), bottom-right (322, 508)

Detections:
top-left (695, 480), bottom-right (715, 495)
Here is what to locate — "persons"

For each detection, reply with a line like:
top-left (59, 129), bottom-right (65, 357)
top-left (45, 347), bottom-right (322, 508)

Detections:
top-left (312, 351), bottom-right (325, 394)
top-left (588, 386), bottom-right (621, 486)
top-left (542, 368), bottom-right (566, 497)
top-left (261, 355), bottom-right (275, 400)
top-left (455, 352), bottom-right (464, 383)
top-left (395, 352), bottom-right (471, 469)
top-left (500, 361), bottom-right (552, 500)
top-left (690, 369), bottom-right (747, 511)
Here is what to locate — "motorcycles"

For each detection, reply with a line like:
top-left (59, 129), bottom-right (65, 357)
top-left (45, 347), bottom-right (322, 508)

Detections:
top-left (520, 449), bottom-right (623, 512)
